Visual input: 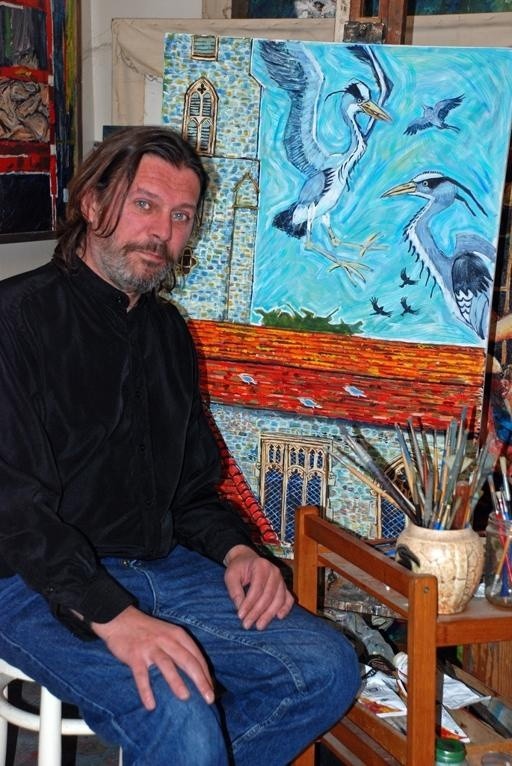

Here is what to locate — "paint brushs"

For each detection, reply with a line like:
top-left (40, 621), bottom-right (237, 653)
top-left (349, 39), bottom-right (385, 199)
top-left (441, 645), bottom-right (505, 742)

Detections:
top-left (327, 406), bottom-right (497, 530)
top-left (485, 456), bottom-right (512, 605)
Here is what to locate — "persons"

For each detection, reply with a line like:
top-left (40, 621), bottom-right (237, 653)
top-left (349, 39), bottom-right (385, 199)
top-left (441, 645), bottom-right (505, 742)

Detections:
top-left (0, 125), bottom-right (360, 766)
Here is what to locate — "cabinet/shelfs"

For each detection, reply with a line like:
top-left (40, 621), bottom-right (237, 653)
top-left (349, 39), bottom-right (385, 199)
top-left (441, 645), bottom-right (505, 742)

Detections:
top-left (282, 503), bottom-right (511, 764)
